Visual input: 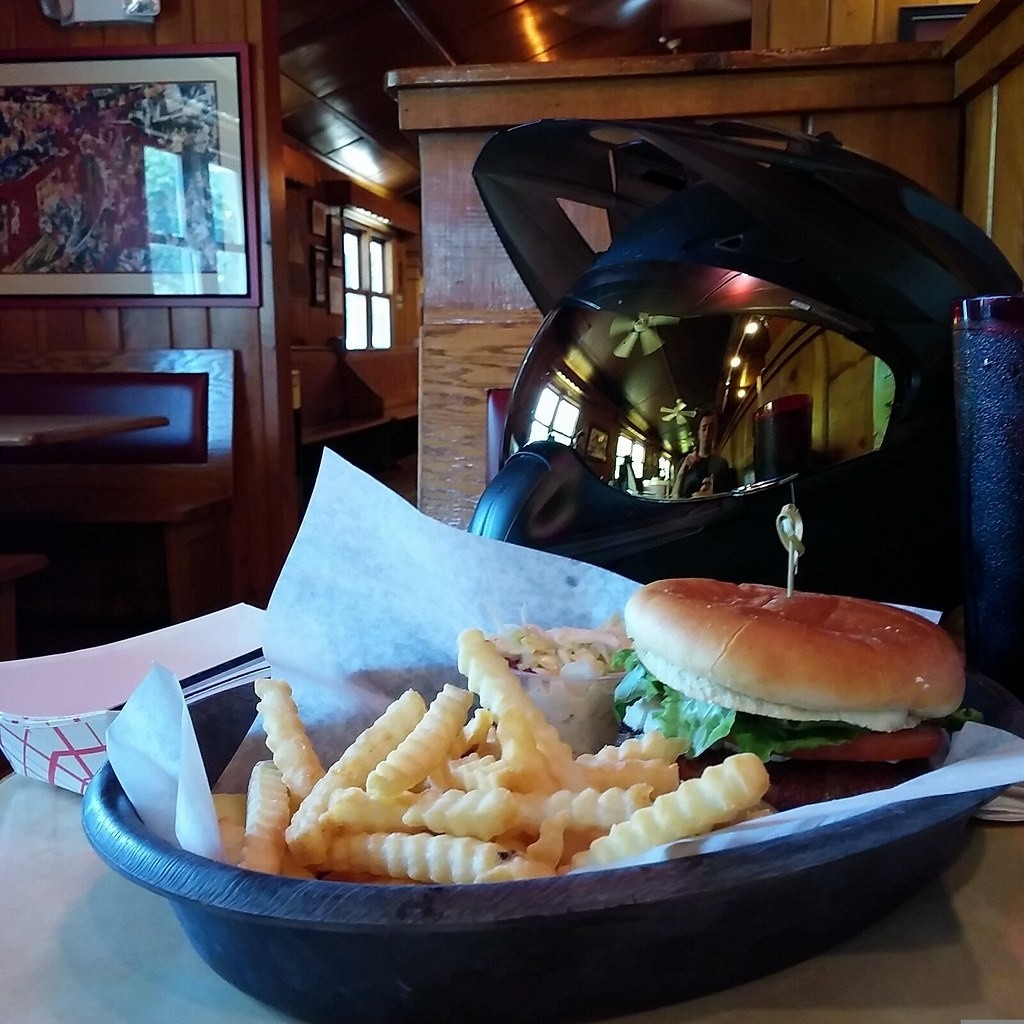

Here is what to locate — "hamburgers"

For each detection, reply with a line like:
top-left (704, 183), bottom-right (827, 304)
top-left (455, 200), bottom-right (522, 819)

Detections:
top-left (607, 578), bottom-right (966, 810)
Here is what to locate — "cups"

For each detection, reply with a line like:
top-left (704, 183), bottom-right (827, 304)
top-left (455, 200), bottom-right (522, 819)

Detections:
top-left (750, 392), bottom-right (813, 484)
top-left (953, 295), bottom-right (1024, 682)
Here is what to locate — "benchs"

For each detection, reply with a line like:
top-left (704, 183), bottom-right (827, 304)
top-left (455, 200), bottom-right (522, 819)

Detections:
top-left (0, 348), bottom-right (235, 604)
top-left (300, 345), bottom-right (417, 446)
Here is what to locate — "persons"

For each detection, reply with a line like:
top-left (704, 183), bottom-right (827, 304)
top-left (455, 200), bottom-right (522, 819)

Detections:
top-left (673, 409), bottom-right (732, 498)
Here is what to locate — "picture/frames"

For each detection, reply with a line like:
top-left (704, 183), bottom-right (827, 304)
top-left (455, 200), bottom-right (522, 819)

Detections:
top-left (0, 43), bottom-right (262, 309)
top-left (586, 422), bottom-right (610, 464)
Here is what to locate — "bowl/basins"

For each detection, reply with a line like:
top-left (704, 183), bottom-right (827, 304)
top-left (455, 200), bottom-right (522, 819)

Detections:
top-left (79, 715), bottom-right (1024, 1024)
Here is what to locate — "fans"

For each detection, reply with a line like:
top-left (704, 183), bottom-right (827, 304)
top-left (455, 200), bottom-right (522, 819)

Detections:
top-left (609, 311), bottom-right (680, 359)
top-left (659, 398), bottom-right (698, 425)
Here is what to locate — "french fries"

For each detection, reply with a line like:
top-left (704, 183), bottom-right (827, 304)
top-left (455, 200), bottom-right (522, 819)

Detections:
top-left (211, 628), bottom-right (775, 885)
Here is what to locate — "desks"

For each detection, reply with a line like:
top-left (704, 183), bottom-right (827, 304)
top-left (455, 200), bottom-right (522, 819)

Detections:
top-left (0, 597), bottom-right (1022, 1024)
top-left (0, 554), bottom-right (49, 660)
top-left (0, 416), bottom-right (171, 475)
